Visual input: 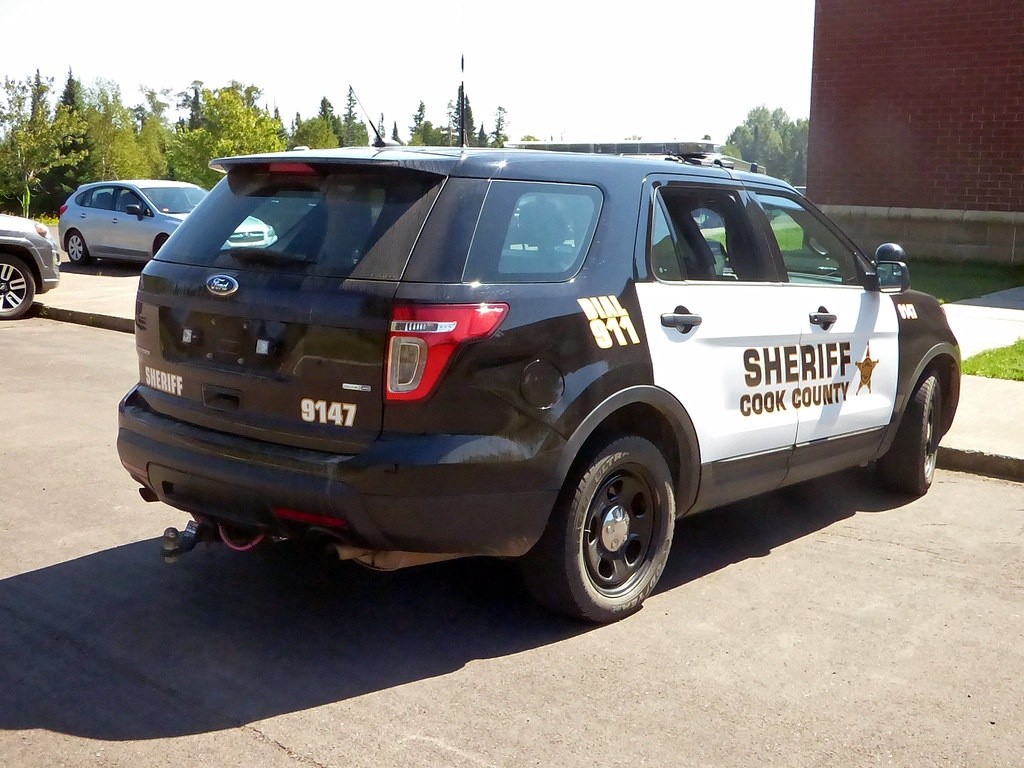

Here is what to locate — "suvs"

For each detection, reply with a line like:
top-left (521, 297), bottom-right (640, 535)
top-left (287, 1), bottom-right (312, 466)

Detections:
top-left (116, 139), bottom-right (962, 629)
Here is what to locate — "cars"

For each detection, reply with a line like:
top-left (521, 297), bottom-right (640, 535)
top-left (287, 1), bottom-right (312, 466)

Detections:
top-left (249, 196), bottom-right (318, 239)
top-left (57, 178), bottom-right (278, 267)
top-left (0, 213), bottom-right (61, 321)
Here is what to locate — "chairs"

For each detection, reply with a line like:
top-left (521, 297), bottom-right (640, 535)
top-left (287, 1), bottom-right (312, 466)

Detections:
top-left (515, 205), bottom-right (574, 264)
top-left (95, 191), bottom-right (111, 208)
